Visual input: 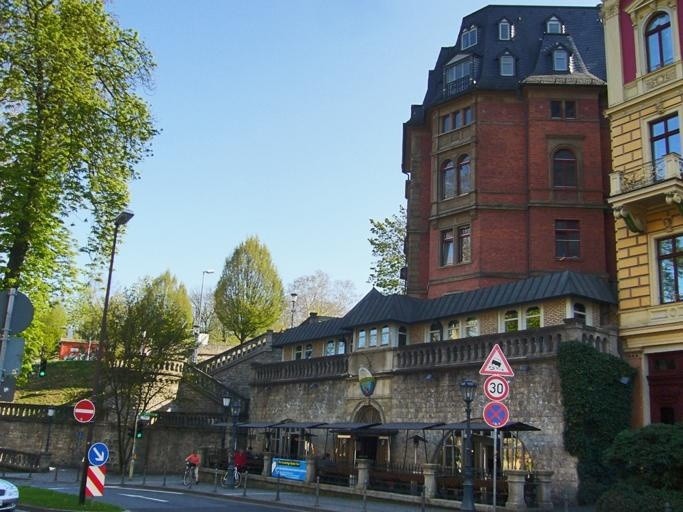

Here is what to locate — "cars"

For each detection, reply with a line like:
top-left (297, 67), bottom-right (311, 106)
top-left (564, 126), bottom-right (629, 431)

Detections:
top-left (0, 478), bottom-right (19, 512)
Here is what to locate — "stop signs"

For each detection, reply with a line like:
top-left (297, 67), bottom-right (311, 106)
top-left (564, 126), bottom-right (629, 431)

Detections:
top-left (72, 398), bottom-right (95, 423)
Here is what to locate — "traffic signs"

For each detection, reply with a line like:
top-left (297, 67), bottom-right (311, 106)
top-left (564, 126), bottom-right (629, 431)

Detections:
top-left (483, 375), bottom-right (509, 401)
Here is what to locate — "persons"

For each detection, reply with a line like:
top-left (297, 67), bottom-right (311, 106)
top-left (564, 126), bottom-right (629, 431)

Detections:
top-left (184, 449), bottom-right (201, 485)
top-left (230, 446), bottom-right (248, 484)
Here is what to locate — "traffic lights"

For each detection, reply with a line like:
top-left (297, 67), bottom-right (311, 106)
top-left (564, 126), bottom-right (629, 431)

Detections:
top-left (38, 357), bottom-right (46, 377)
top-left (134, 418), bottom-right (145, 440)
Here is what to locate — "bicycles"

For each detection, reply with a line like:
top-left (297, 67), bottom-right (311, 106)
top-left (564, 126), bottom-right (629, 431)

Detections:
top-left (219, 463), bottom-right (242, 488)
top-left (182, 460), bottom-right (197, 486)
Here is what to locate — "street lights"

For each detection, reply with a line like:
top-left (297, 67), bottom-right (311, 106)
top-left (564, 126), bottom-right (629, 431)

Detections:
top-left (289, 293), bottom-right (298, 328)
top-left (457, 374), bottom-right (478, 512)
top-left (219, 391), bottom-right (232, 468)
top-left (38, 404), bottom-right (57, 454)
top-left (223, 400), bottom-right (241, 489)
top-left (76, 208), bottom-right (135, 503)
top-left (198, 270), bottom-right (214, 330)
